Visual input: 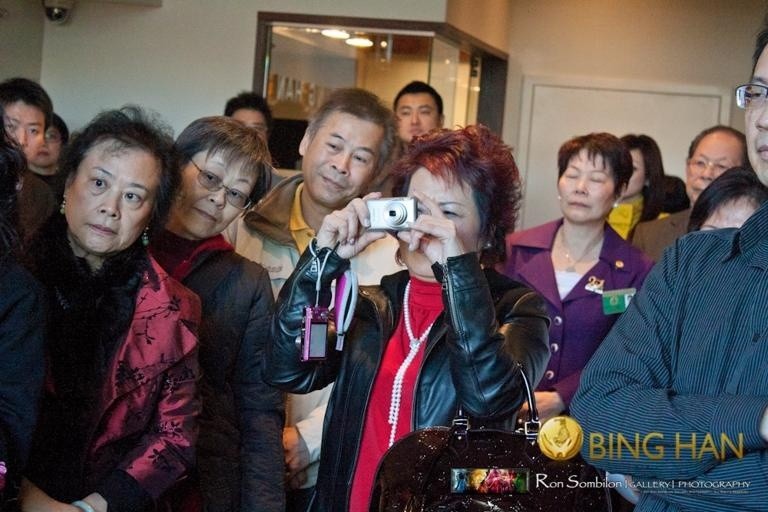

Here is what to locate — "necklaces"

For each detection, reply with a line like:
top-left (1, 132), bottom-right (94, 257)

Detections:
top-left (387, 278), bottom-right (433, 448)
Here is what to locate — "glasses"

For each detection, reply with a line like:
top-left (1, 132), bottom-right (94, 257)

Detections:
top-left (735, 83), bottom-right (767, 110)
top-left (188, 156), bottom-right (252, 212)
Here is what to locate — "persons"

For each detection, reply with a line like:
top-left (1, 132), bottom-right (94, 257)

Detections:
top-left (495, 15), bottom-right (768, 512)
top-left (222, 94), bottom-right (285, 171)
top-left (142, 113), bottom-right (294, 512)
top-left (263, 122), bottom-right (550, 511)
top-left (367, 76), bottom-right (449, 197)
top-left (0, 78), bottom-right (54, 169)
top-left (0, 105), bottom-right (49, 511)
top-left (209, 88), bottom-right (407, 512)
top-left (16, 103), bottom-right (207, 511)
top-left (23, 114), bottom-right (80, 206)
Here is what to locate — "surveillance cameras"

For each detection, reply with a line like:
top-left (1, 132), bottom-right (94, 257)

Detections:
top-left (43, 0), bottom-right (74, 24)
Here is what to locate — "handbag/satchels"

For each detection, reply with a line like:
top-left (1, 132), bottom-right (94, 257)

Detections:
top-left (369, 366), bottom-right (630, 512)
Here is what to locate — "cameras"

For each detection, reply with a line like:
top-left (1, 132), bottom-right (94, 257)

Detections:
top-left (367, 198), bottom-right (417, 232)
top-left (295, 306), bottom-right (329, 363)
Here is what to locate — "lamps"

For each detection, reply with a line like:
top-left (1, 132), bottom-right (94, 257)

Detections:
top-left (344, 33), bottom-right (374, 47)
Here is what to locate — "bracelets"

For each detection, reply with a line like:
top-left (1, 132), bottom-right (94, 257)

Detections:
top-left (69, 497), bottom-right (99, 512)
